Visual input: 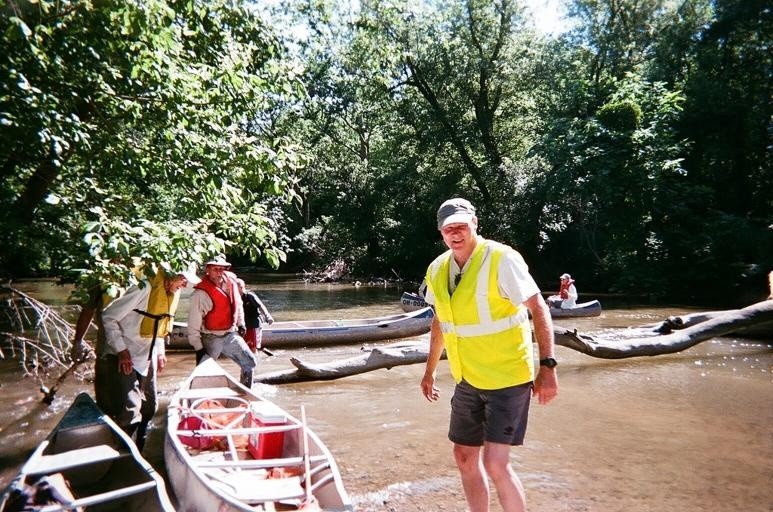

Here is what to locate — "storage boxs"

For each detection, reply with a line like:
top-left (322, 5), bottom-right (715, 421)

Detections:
top-left (245, 401), bottom-right (288, 460)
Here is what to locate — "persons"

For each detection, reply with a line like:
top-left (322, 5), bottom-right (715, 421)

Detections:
top-left (101, 259), bottom-right (202, 455)
top-left (235, 278), bottom-right (274, 354)
top-left (71, 254), bottom-right (147, 420)
top-left (544, 272), bottom-right (578, 310)
top-left (185, 252), bottom-right (255, 390)
top-left (419, 199), bottom-right (560, 512)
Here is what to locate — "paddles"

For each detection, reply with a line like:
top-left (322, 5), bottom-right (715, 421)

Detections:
top-left (297, 405), bottom-right (319, 512)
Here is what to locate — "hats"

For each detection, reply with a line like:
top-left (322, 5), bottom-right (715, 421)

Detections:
top-left (437, 198), bottom-right (473, 230)
top-left (560, 274), bottom-right (570, 279)
top-left (180, 263), bottom-right (202, 284)
top-left (204, 253), bottom-right (232, 266)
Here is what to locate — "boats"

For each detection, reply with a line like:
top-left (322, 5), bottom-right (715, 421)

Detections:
top-left (2, 392), bottom-right (178, 512)
top-left (166, 306), bottom-right (437, 351)
top-left (163, 357), bottom-right (357, 512)
top-left (399, 291), bottom-right (602, 320)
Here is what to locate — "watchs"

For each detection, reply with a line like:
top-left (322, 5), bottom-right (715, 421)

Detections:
top-left (539, 357), bottom-right (557, 369)
